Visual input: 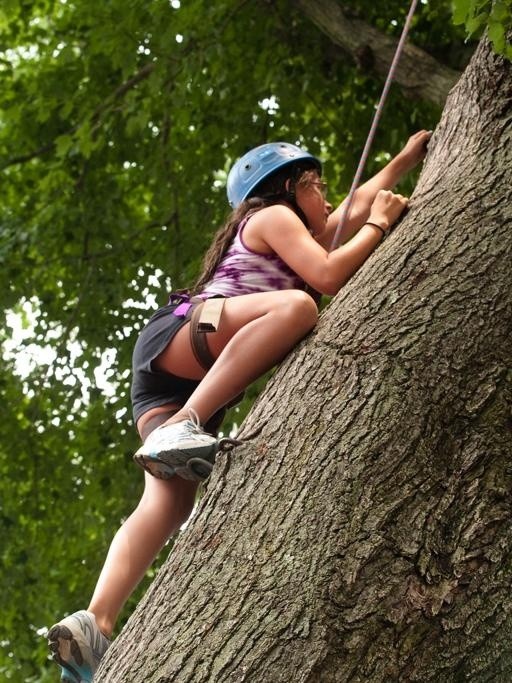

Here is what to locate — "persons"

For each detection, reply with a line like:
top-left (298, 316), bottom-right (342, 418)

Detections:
top-left (48, 128), bottom-right (434, 683)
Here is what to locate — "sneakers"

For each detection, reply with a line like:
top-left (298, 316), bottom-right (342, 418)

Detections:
top-left (132, 419), bottom-right (219, 482)
top-left (46, 610), bottom-right (113, 683)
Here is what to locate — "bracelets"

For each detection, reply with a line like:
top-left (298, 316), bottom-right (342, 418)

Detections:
top-left (364, 222), bottom-right (385, 236)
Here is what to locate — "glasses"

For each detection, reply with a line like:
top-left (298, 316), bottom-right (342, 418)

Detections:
top-left (310, 179), bottom-right (327, 197)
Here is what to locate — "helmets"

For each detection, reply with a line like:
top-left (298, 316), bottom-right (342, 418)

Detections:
top-left (226, 142), bottom-right (324, 211)
